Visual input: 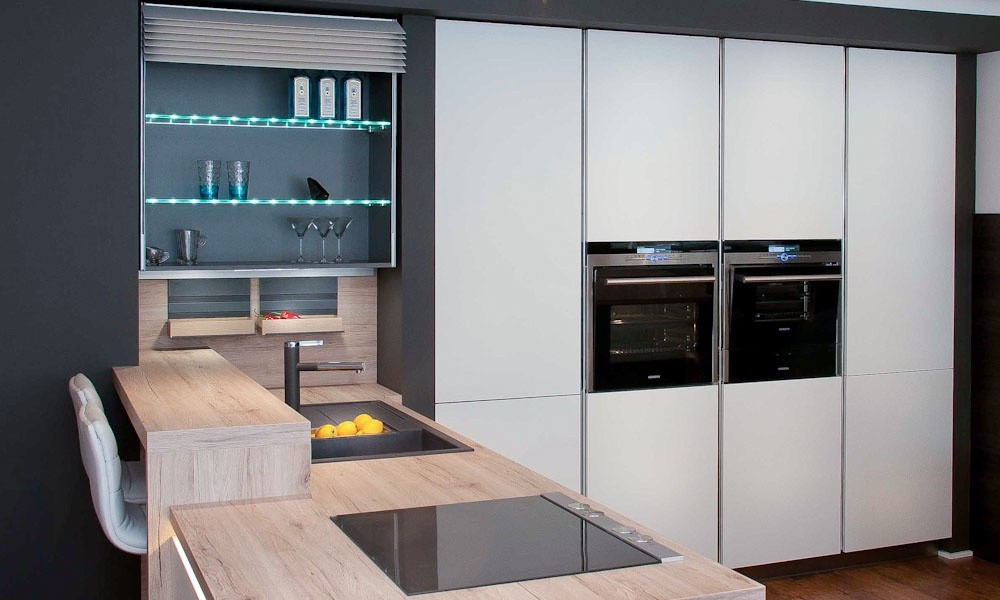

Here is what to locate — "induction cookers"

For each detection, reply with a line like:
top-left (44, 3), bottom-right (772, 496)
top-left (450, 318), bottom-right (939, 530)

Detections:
top-left (330, 491), bottom-right (685, 596)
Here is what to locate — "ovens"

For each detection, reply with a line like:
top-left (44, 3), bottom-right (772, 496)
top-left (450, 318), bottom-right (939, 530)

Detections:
top-left (584, 239), bottom-right (844, 394)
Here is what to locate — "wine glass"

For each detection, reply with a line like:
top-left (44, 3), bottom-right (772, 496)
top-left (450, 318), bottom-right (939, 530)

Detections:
top-left (287, 217), bottom-right (314, 263)
top-left (328, 217), bottom-right (354, 262)
top-left (310, 217), bottom-right (338, 263)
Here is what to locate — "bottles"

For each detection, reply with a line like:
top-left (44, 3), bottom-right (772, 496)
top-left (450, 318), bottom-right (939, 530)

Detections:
top-left (287, 68), bottom-right (363, 120)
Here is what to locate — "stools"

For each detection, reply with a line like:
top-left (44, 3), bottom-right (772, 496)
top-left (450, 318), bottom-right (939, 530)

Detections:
top-left (69, 373), bottom-right (149, 600)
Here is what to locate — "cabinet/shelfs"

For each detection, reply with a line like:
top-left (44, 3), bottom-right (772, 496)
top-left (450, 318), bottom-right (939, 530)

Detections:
top-left (137, 3), bottom-right (407, 279)
top-left (379, 11), bottom-right (974, 572)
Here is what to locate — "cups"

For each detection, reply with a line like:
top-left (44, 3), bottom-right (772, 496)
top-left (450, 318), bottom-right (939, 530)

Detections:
top-left (196, 160), bottom-right (221, 199)
top-left (226, 160), bottom-right (250, 199)
top-left (175, 229), bottom-right (207, 266)
top-left (145, 247), bottom-right (170, 266)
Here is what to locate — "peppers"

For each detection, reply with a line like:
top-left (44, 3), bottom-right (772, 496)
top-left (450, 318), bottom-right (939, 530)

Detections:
top-left (253, 309), bottom-right (300, 320)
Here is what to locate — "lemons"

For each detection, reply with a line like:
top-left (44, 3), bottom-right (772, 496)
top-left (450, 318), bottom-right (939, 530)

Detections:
top-left (314, 414), bottom-right (384, 437)
